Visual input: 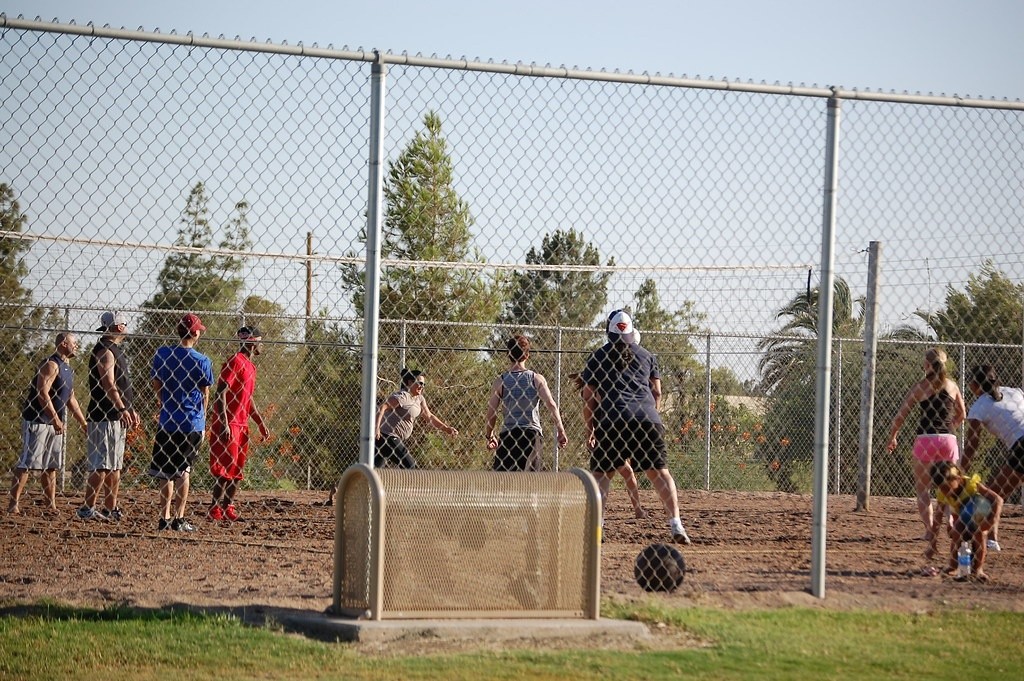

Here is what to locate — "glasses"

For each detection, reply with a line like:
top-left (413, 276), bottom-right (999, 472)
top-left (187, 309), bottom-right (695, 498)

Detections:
top-left (123, 322), bottom-right (127, 326)
top-left (417, 382), bottom-right (426, 386)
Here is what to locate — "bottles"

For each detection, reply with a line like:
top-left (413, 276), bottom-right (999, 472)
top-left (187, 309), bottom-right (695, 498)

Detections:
top-left (957, 542), bottom-right (971, 578)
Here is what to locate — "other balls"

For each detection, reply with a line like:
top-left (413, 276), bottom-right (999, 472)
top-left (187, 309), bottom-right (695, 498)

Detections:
top-left (958, 495), bottom-right (993, 527)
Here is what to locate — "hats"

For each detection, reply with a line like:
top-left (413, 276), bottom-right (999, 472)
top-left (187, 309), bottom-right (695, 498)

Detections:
top-left (606, 310), bottom-right (635, 343)
top-left (176, 314), bottom-right (206, 331)
top-left (633, 328), bottom-right (640, 345)
top-left (95, 311), bottom-right (125, 331)
top-left (237, 326), bottom-right (261, 338)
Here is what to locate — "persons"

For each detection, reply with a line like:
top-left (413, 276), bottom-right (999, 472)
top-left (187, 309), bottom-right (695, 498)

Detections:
top-left (960, 364), bottom-right (1024, 482)
top-left (486, 336), bottom-right (569, 506)
top-left (8, 333), bottom-right (87, 517)
top-left (569, 368), bottom-right (647, 520)
top-left (582, 309), bottom-right (691, 544)
top-left (374, 369), bottom-right (459, 470)
top-left (76, 311), bottom-right (141, 521)
top-left (149, 314), bottom-right (215, 533)
top-left (886, 348), bottom-right (967, 539)
top-left (210, 325), bottom-right (270, 521)
top-left (921, 460), bottom-right (1004, 578)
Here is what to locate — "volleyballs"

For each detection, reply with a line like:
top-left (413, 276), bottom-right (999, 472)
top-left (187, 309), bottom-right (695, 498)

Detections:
top-left (633, 327), bottom-right (641, 344)
top-left (634, 543), bottom-right (685, 593)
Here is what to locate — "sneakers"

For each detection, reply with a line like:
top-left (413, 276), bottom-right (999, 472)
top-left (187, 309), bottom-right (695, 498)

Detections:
top-left (224, 505), bottom-right (245, 521)
top-left (76, 507), bottom-right (110, 523)
top-left (101, 507), bottom-right (125, 521)
top-left (671, 525), bottom-right (690, 544)
top-left (171, 516), bottom-right (198, 532)
top-left (159, 518), bottom-right (182, 531)
top-left (207, 506), bottom-right (224, 521)
